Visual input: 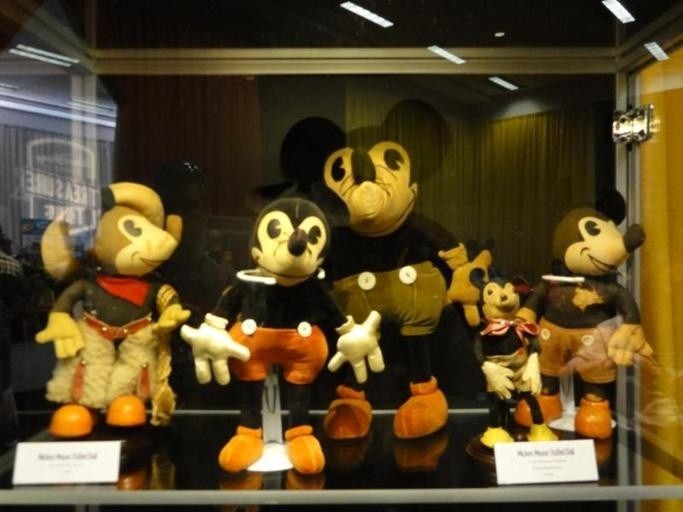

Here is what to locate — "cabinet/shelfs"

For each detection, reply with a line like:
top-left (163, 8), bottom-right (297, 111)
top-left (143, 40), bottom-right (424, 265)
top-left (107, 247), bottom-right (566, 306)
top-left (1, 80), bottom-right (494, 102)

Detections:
top-left (0, 1), bottom-right (683, 512)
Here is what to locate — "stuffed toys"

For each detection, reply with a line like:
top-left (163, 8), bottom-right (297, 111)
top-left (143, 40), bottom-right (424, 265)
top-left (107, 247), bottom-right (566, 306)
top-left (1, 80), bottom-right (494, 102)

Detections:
top-left (464, 261), bottom-right (560, 450)
top-left (277, 95), bottom-right (496, 448)
top-left (174, 195), bottom-right (387, 476)
top-left (31, 182), bottom-right (192, 441)
top-left (513, 187), bottom-right (655, 442)
